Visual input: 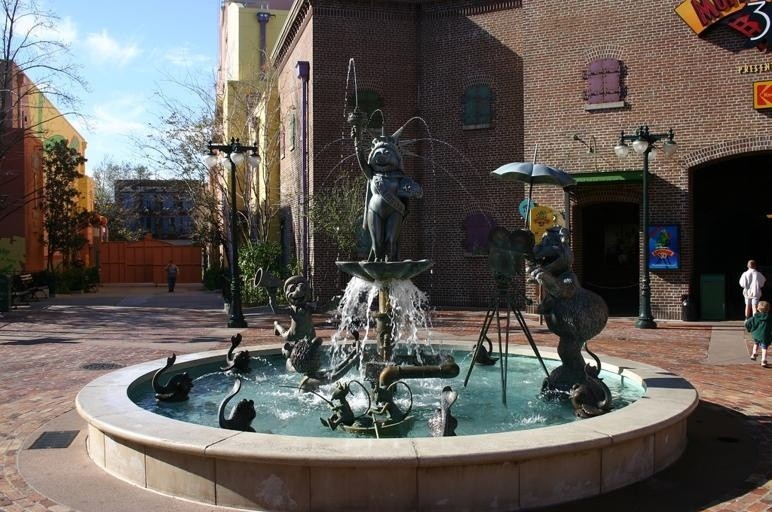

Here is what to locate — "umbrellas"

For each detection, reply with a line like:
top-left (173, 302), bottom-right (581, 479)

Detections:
top-left (489, 143), bottom-right (577, 231)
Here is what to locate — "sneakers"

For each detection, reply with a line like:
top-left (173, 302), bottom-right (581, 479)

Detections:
top-left (761, 360), bottom-right (768, 367)
top-left (751, 354), bottom-right (757, 360)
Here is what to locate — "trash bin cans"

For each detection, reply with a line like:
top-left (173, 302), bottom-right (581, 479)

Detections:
top-left (0, 273), bottom-right (11, 312)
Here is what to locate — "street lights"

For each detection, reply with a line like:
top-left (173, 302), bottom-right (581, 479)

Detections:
top-left (201, 135), bottom-right (264, 327)
top-left (610, 120), bottom-right (679, 331)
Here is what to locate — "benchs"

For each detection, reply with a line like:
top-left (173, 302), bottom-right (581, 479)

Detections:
top-left (10, 274), bottom-right (48, 305)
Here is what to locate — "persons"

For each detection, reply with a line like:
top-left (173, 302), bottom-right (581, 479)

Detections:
top-left (744, 301), bottom-right (772, 366)
top-left (163, 259), bottom-right (178, 293)
top-left (738, 260), bottom-right (766, 322)
